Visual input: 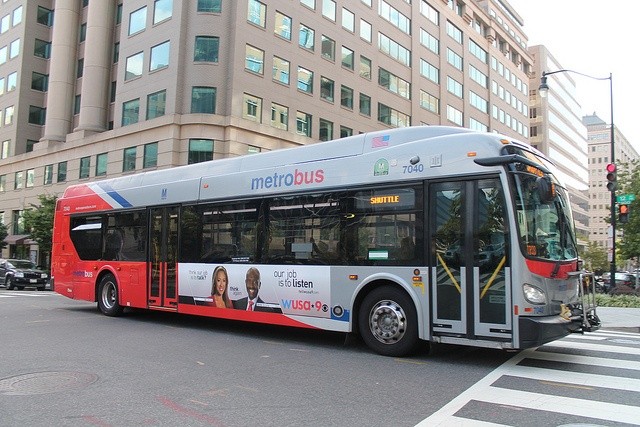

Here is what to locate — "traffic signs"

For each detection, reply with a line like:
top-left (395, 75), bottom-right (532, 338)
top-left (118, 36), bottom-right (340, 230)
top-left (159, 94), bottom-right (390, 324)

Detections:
top-left (615, 193), bottom-right (635, 205)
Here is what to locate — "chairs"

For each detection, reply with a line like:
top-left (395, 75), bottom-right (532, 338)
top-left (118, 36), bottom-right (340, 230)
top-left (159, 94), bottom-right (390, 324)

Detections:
top-left (401, 237), bottom-right (416, 252)
top-left (318, 239), bottom-right (331, 251)
top-left (331, 239), bottom-right (339, 250)
top-left (293, 238), bottom-right (305, 244)
top-left (268, 237), bottom-right (285, 258)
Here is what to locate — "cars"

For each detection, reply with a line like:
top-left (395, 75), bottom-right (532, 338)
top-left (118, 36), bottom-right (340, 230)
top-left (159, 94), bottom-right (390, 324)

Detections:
top-left (600, 272), bottom-right (636, 292)
top-left (0, 258), bottom-right (50, 293)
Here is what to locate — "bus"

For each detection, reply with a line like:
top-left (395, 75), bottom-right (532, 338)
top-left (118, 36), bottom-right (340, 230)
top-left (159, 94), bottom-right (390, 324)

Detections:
top-left (39, 123), bottom-right (602, 358)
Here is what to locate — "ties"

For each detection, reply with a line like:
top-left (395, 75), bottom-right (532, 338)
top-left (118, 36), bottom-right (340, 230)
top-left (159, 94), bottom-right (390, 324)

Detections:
top-left (248, 301), bottom-right (254, 311)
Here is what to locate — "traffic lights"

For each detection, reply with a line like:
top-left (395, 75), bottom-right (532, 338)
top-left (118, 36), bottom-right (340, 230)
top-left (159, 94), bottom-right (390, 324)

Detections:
top-left (606, 163), bottom-right (617, 192)
top-left (619, 203), bottom-right (629, 223)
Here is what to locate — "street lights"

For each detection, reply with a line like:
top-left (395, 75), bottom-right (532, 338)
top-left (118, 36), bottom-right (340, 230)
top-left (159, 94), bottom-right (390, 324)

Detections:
top-left (537, 70), bottom-right (621, 292)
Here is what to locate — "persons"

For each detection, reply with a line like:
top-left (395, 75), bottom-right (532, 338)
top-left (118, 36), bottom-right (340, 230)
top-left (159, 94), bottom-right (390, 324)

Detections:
top-left (202, 264), bottom-right (234, 309)
top-left (233, 266), bottom-right (274, 312)
